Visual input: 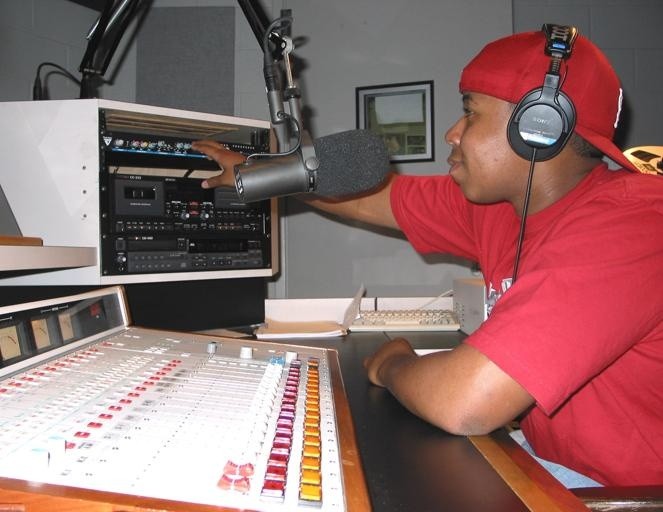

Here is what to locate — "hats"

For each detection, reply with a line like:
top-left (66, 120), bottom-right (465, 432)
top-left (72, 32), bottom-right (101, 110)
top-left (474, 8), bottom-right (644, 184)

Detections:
top-left (460, 30), bottom-right (640, 174)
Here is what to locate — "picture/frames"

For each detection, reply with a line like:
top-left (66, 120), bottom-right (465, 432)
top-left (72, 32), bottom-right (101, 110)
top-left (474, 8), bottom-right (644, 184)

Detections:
top-left (356, 79), bottom-right (436, 162)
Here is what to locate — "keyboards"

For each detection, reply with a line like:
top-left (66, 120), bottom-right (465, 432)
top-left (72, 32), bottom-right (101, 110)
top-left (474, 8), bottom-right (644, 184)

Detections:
top-left (349, 309), bottom-right (460, 332)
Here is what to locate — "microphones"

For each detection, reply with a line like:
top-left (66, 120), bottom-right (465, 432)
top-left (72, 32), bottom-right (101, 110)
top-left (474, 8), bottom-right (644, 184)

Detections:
top-left (234, 130), bottom-right (392, 202)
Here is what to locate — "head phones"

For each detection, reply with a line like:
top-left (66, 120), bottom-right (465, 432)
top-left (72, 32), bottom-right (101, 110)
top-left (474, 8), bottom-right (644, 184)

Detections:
top-left (507, 21), bottom-right (579, 164)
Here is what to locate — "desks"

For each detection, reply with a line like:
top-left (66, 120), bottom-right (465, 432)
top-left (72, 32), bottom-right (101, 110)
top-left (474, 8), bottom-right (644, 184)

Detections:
top-left (247, 329), bottom-right (589, 512)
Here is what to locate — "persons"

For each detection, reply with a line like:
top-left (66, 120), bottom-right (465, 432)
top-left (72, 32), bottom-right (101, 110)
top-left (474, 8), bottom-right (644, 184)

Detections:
top-left (187, 28), bottom-right (663, 490)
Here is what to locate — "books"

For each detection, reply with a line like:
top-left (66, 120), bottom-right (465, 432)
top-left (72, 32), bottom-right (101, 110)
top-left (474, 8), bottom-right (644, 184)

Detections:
top-left (254, 320), bottom-right (347, 339)
